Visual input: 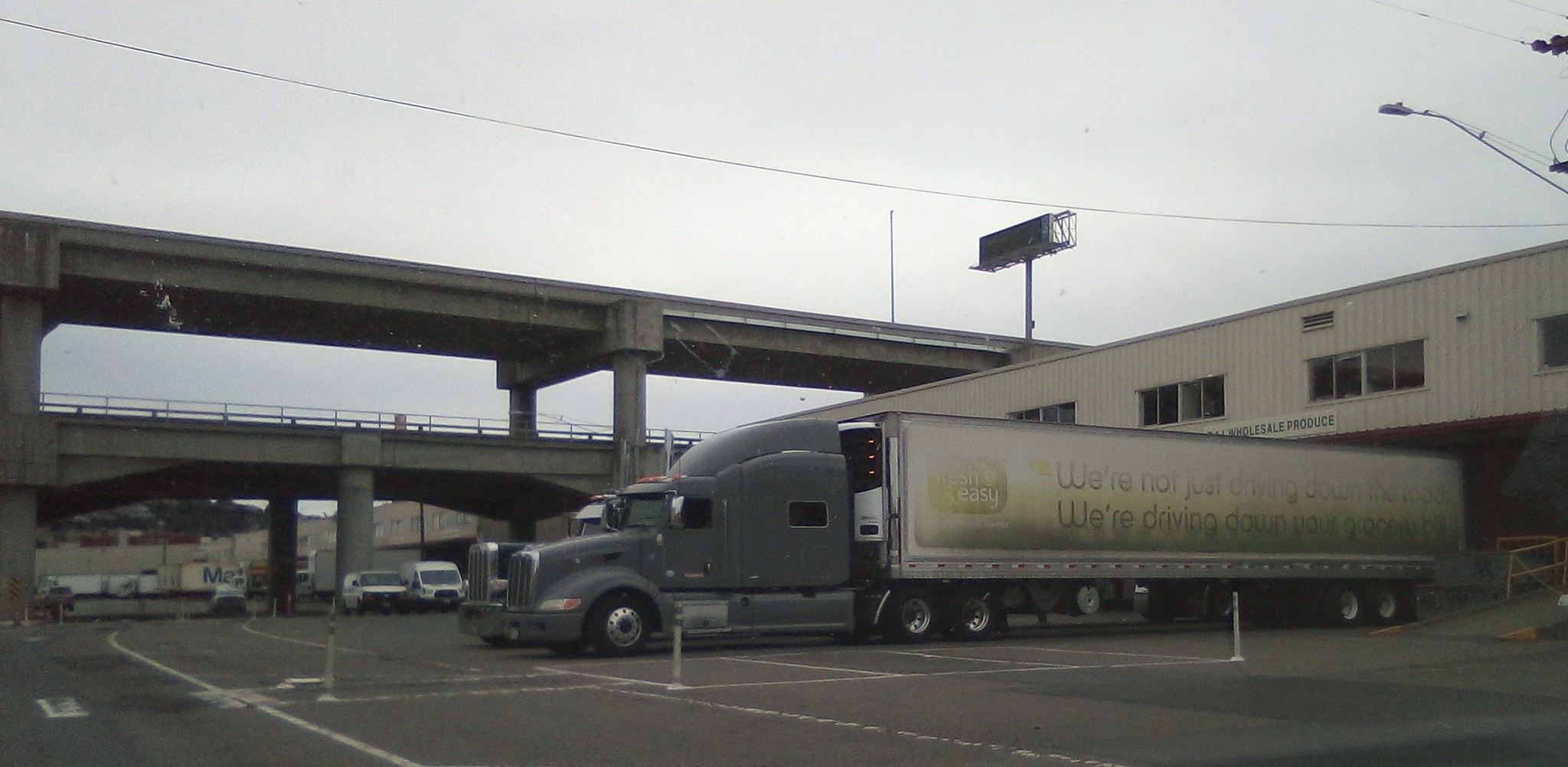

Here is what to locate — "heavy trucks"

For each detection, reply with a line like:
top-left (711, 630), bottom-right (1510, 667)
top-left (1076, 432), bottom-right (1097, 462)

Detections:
top-left (458, 410), bottom-right (1470, 658)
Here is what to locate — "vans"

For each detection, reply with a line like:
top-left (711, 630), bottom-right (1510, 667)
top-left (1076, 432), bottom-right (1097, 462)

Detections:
top-left (340, 571), bottom-right (410, 616)
top-left (397, 563), bottom-right (466, 614)
